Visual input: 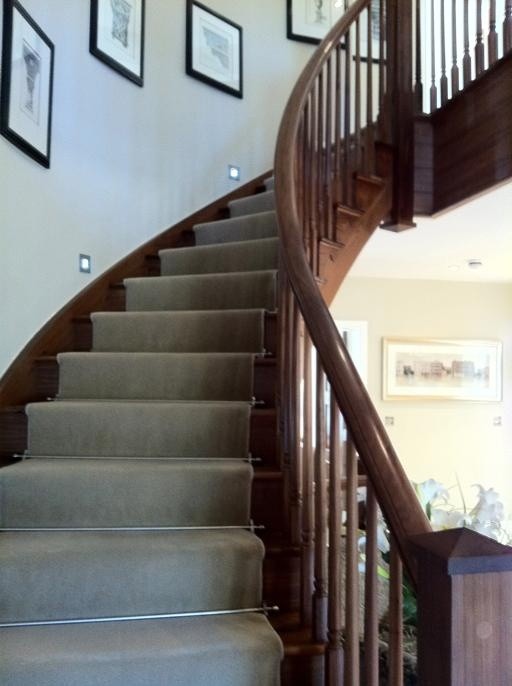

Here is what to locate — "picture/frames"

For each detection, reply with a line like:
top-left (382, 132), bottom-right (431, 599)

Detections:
top-left (1, 0), bottom-right (54, 170)
top-left (89, 0), bottom-right (145, 89)
top-left (381, 334), bottom-right (505, 402)
top-left (287, 2), bottom-right (387, 62)
top-left (185, 1), bottom-right (245, 99)
